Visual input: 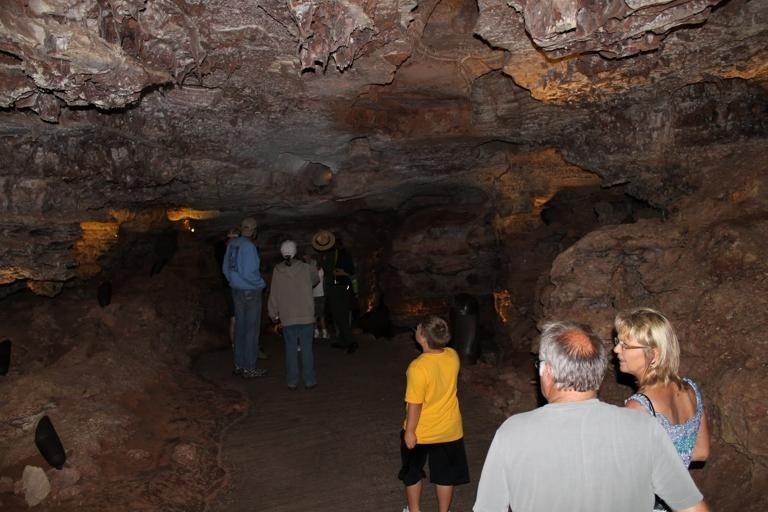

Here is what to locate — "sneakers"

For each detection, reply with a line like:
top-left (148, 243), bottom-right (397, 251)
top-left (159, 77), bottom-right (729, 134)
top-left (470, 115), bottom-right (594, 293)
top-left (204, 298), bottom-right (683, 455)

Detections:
top-left (286, 381), bottom-right (317, 390)
top-left (230, 357), bottom-right (269, 378)
top-left (313, 328), bottom-right (358, 354)
top-left (403, 505), bottom-right (409, 512)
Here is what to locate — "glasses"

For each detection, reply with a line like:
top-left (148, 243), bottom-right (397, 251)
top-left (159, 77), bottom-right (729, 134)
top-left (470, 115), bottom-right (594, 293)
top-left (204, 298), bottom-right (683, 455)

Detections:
top-left (613, 336), bottom-right (656, 351)
top-left (534, 359), bottom-right (546, 370)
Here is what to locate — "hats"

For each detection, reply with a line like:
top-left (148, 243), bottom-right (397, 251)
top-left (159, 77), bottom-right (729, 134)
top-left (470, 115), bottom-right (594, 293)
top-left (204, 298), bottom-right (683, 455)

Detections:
top-left (311, 230), bottom-right (336, 251)
top-left (279, 240), bottom-right (298, 259)
top-left (240, 217), bottom-right (258, 237)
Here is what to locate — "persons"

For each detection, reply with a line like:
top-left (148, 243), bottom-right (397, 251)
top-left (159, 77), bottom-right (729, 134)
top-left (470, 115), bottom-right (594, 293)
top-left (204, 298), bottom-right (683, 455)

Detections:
top-left (302, 245), bottom-right (328, 339)
top-left (266, 238), bottom-right (321, 392)
top-left (398, 313), bottom-right (470, 512)
top-left (220, 224), bottom-right (241, 353)
top-left (314, 230), bottom-right (359, 355)
top-left (612, 308), bottom-right (711, 470)
top-left (221, 218), bottom-right (271, 379)
top-left (472, 319), bottom-right (710, 512)
